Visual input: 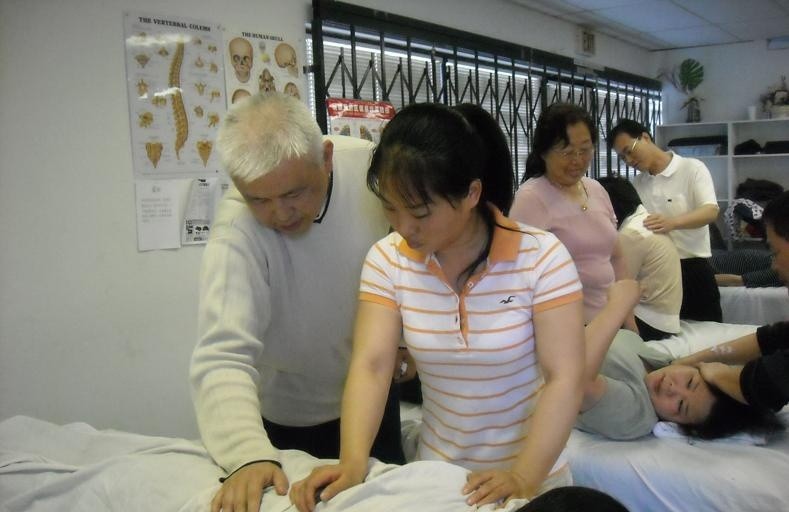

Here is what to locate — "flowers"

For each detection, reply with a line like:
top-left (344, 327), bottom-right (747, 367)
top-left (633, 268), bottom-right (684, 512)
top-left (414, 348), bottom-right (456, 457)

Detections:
top-left (760, 71), bottom-right (789, 112)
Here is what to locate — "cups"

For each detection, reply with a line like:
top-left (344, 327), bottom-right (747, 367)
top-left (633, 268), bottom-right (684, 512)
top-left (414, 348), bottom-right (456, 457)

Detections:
top-left (746, 106), bottom-right (757, 120)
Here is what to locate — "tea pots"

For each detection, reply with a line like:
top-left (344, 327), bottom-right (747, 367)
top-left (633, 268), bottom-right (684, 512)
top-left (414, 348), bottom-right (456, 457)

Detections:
top-left (768, 89), bottom-right (789, 120)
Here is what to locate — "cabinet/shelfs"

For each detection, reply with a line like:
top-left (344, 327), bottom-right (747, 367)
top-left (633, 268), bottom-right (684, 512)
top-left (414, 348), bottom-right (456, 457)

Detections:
top-left (654, 117), bottom-right (789, 251)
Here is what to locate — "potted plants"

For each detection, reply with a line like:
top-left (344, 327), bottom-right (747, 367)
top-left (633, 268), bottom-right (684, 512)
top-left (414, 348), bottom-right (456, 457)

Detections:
top-left (654, 58), bottom-right (704, 122)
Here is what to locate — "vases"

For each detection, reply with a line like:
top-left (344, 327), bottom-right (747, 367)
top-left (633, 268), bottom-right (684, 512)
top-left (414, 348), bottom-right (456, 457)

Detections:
top-left (768, 104), bottom-right (789, 119)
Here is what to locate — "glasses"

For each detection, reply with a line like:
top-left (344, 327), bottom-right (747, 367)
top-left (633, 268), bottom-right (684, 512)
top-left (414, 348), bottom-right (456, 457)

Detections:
top-left (617, 136), bottom-right (641, 163)
top-left (550, 146), bottom-right (596, 162)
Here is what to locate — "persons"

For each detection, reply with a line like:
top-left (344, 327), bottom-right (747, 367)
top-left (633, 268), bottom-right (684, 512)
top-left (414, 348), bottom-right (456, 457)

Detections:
top-left (670, 188), bottom-right (789, 414)
top-left (605, 118), bottom-right (723, 322)
top-left (290, 103), bottom-right (587, 512)
top-left (399, 328), bottom-right (785, 443)
top-left (506, 102), bottom-right (619, 325)
top-left (593, 177), bottom-right (683, 341)
top-left (187, 88), bottom-right (417, 512)
top-left (709, 249), bottom-right (784, 287)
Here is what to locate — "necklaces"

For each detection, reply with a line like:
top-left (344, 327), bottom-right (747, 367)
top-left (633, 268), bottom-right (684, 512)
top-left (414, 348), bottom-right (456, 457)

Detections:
top-left (580, 204), bottom-right (588, 211)
